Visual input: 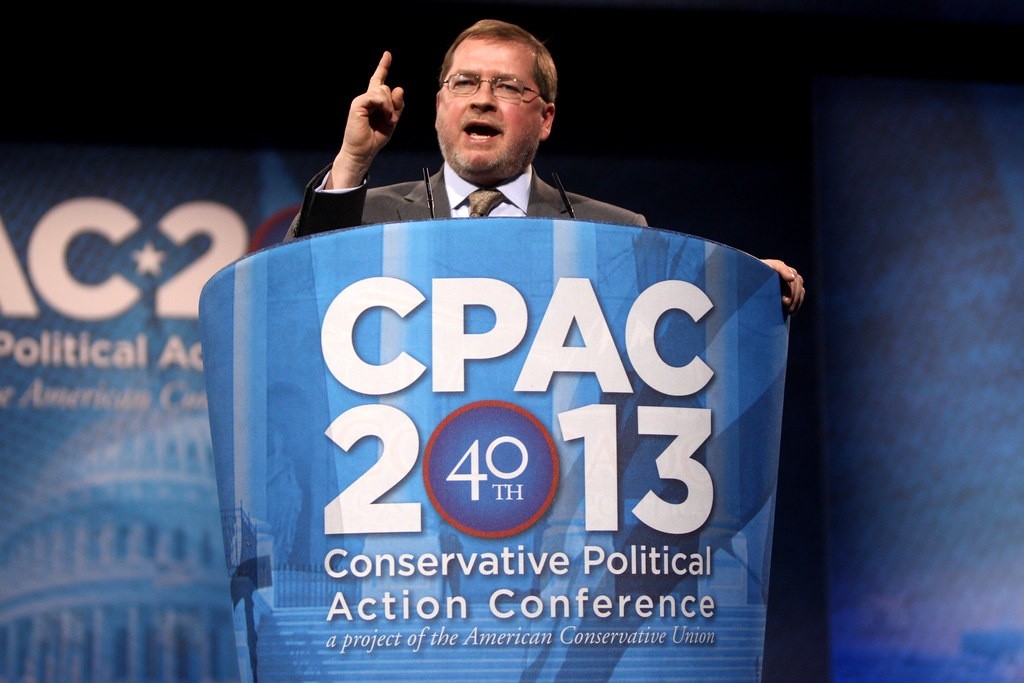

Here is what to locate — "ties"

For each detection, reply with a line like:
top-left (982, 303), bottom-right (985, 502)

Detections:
top-left (467, 189), bottom-right (505, 218)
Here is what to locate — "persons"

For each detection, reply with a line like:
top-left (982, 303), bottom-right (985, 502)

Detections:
top-left (285, 19), bottom-right (806, 310)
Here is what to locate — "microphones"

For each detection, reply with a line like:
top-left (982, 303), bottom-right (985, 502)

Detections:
top-left (552, 172), bottom-right (575, 219)
top-left (422, 167), bottom-right (436, 219)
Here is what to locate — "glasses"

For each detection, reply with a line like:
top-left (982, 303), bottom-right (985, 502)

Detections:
top-left (442, 73), bottom-right (546, 99)
